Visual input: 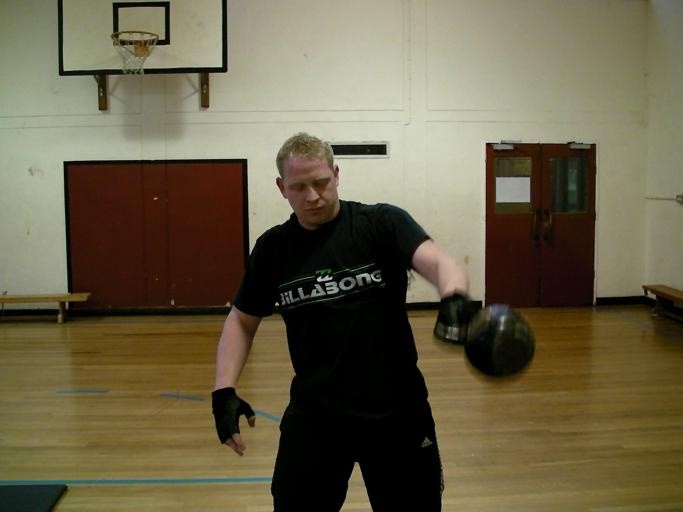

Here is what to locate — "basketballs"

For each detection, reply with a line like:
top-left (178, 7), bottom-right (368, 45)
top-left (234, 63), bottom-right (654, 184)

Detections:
top-left (464, 305), bottom-right (533, 378)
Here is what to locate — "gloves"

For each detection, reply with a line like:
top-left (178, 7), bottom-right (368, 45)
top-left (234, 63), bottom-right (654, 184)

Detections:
top-left (209, 386), bottom-right (255, 445)
top-left (431, 296), bottom-right (485, 345)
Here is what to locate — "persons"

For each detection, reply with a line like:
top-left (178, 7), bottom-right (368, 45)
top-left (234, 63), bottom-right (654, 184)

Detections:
top-left (209, 131), bottom-right (472, 512)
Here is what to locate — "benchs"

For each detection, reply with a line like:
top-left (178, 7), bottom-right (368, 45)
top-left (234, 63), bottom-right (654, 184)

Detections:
top-left (641, 281), bottom-right (682, 323)
top-left (1, 293), bottom-right (92, 324)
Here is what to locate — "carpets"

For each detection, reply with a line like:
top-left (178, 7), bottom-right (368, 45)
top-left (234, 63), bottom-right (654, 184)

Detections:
top-left (0, 484), bottom-right (68, 511)
